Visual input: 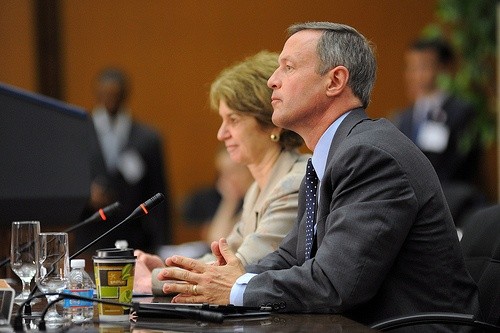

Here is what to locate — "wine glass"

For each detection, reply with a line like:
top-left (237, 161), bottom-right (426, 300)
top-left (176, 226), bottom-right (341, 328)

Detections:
top-left (10, 221), bottom-right (69, 320)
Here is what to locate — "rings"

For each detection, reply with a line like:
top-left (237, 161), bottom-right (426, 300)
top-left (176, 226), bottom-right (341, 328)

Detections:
top-left (193, 284), bottom-right (198, 295)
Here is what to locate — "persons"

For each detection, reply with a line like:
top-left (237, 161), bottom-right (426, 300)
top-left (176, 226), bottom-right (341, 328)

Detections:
top-left (128, 50), bottom-right (312, 296)
top-left (186, 151), bottom-right (254, 245)
top-left (76, 65), bottom-right (171, 253)
top-left (158, 21), bottom-right (480, 333)
top-left (393, 35), bottom-right (485, 223)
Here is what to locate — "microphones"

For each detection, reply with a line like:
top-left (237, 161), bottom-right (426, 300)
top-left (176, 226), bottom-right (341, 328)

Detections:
top-left (1, 201), bottom-right (119, 270)
top-left (12, 292), bottom-right (224, 333)
top-left (24, 191), bottom-right (166, 321)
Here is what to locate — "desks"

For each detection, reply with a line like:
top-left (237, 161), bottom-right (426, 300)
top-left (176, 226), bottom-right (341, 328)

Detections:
top-left (0, 295), bottom-right (383, 333)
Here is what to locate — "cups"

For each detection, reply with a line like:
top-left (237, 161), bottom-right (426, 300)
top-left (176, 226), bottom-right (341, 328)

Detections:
top-left (92, 248), bottom-right (138, 322)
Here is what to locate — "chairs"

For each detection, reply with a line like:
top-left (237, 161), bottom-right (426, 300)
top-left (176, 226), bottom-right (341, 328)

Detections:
top-left (370, 179), bottom-right (500, 333)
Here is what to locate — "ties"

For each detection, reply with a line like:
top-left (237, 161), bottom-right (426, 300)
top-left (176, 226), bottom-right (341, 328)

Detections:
top-left (305, 158), bottom-right (317, 262)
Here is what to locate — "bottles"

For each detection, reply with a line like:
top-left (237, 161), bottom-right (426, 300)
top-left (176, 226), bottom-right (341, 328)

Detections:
top-left (63, 259), bottom-right (95, 321)
